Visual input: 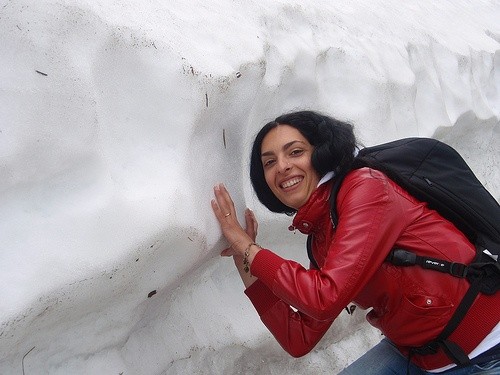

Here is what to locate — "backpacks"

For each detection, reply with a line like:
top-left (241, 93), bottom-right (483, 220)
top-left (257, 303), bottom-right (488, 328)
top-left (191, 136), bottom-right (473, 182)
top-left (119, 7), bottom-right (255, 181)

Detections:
top-left (306, 137), bottom-right (500, 368)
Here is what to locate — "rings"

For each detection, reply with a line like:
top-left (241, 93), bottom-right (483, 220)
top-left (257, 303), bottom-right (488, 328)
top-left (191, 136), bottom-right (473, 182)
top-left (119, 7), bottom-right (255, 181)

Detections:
top-left (225, 213), bottom-right (232, 217)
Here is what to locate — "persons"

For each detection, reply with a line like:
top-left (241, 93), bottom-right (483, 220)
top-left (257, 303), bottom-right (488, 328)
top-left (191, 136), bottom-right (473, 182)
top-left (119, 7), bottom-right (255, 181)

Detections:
top-left (210, 111), bottom-right (500, 375)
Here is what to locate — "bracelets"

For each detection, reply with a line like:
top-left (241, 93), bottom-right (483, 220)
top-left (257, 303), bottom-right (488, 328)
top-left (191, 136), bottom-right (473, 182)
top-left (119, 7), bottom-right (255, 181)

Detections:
top-left (242, 243), bottom-right (260, 273)
